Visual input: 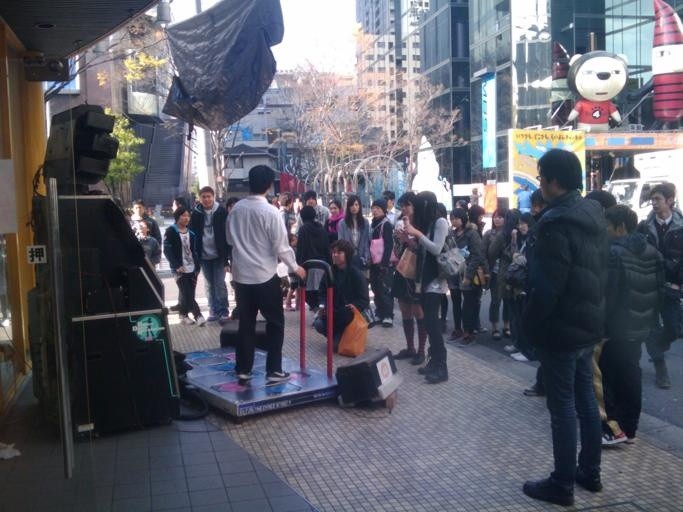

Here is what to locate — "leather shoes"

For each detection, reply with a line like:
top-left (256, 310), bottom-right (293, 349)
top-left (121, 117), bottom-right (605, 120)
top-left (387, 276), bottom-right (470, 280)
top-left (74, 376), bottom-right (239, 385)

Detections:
top-left (655, 366), bottom-right (672, 388)
top-left (411, 355), bottom-right (424, 364)
top-left (522, 385), bottom-right (545, 396)
top-left (391, 350), bottom-right (415, 359)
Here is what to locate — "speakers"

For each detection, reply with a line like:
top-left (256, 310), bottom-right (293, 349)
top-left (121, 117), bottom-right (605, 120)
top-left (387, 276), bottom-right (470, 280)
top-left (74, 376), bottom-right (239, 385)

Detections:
top-left (220, 319), bottom-right (269, 351)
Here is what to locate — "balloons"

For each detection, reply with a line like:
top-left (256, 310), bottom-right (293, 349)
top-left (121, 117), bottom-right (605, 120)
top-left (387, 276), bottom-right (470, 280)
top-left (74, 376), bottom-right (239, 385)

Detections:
top-left (567, 50), bottom-right (628, 132)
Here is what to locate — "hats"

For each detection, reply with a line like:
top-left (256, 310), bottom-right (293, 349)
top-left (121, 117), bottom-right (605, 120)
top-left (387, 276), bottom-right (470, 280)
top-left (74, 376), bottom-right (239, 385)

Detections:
top-left (373, 198), bottom-right (388, 212)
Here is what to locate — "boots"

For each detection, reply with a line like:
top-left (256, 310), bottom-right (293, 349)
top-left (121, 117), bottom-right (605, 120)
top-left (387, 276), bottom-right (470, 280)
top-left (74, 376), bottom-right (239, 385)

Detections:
top-left (424, 351), bottom-right (448, 382)
top-left (418, 348), bottom-right (432, 374)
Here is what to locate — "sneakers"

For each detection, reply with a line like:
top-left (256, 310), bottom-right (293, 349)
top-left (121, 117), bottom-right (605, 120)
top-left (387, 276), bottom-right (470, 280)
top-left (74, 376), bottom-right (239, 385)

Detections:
top-left (374, 316), bottom-right (380, 322)
top-left (266, 371), bottom-right (289, 382)
top-left (445, 326), bottom-right (529, 363)
top-left (601, 430), bottom-right (627, 446)
top-left (575, 466), bottom-right (601, 491)
top-left (181, 314), bottom-right (230, 326)
top-left (523, 477), bottom-right (573, 505)
top-left (170, 305), bottom-right (179, 311)
top-left (382, 317), bottom-right (394, 326)
top-left (238, 372), bottom-right (252, 379)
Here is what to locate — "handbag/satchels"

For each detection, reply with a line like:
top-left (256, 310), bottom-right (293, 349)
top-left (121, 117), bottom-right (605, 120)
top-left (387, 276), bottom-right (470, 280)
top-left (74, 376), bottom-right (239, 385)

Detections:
top-left (368, 219), bottom-right (397, 264)
top-left (395, 248), bottom-right (416, 279)
top-left (436, 246), bottom-right (470, 283)
top-left (501, 261), bottom-right (527, 289)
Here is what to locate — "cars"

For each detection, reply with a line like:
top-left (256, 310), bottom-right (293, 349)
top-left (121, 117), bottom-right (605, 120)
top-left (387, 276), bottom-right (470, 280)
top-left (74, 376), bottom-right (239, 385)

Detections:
top-left (150, 220), bottom-right (175, 279)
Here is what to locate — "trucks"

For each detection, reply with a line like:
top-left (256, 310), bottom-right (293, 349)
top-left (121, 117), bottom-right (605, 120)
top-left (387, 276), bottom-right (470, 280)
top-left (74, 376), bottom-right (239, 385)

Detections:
top-left (608, 175), bottom-right (675, 225)
